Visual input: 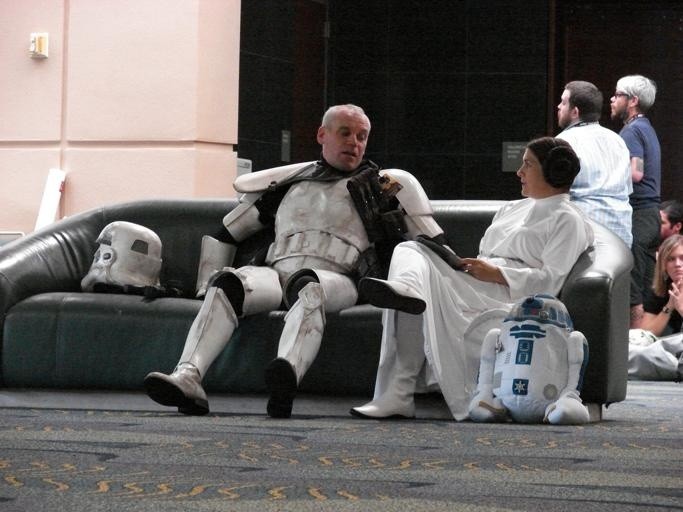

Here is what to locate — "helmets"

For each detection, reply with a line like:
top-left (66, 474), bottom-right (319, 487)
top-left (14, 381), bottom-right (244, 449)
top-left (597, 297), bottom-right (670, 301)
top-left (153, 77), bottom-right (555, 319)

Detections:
top-left (81, 221), bottom-right (167, 292)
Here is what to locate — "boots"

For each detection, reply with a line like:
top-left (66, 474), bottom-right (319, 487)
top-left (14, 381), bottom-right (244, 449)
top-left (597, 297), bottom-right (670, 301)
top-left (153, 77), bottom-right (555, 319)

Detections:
top-left (142, 286), bottom-right (239, 415)
top-left (263, 282), bottom-right (327, 418)
top-left (358, 247), bottom-right (427, 315)
top-left (349, 311), bottom-right (426, 419)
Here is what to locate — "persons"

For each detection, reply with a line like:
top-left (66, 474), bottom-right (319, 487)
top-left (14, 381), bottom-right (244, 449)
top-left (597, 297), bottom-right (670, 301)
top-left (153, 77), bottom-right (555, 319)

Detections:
top-left (553, 79), bottom-right (635, 250)
top-left (350, 136), bottom-right (591, 421)
top-left (625, 233), bottom-right (683, 384)
top-left (653, 199), bottom-right (683, 261)
top-left (142, 104), bottom-right (465, 419)
top-left (609, 73), bottom-right (662, 331)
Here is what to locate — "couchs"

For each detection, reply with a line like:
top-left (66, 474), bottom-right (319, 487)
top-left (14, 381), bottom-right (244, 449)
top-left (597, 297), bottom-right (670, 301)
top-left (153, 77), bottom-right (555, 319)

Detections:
top-left (0, 196), bottom-right (634, 423)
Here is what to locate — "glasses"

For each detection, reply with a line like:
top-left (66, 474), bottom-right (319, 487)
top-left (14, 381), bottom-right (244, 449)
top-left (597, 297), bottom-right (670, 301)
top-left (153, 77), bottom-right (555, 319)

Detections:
top-left (614, 91), bottom-right (628, 99)
